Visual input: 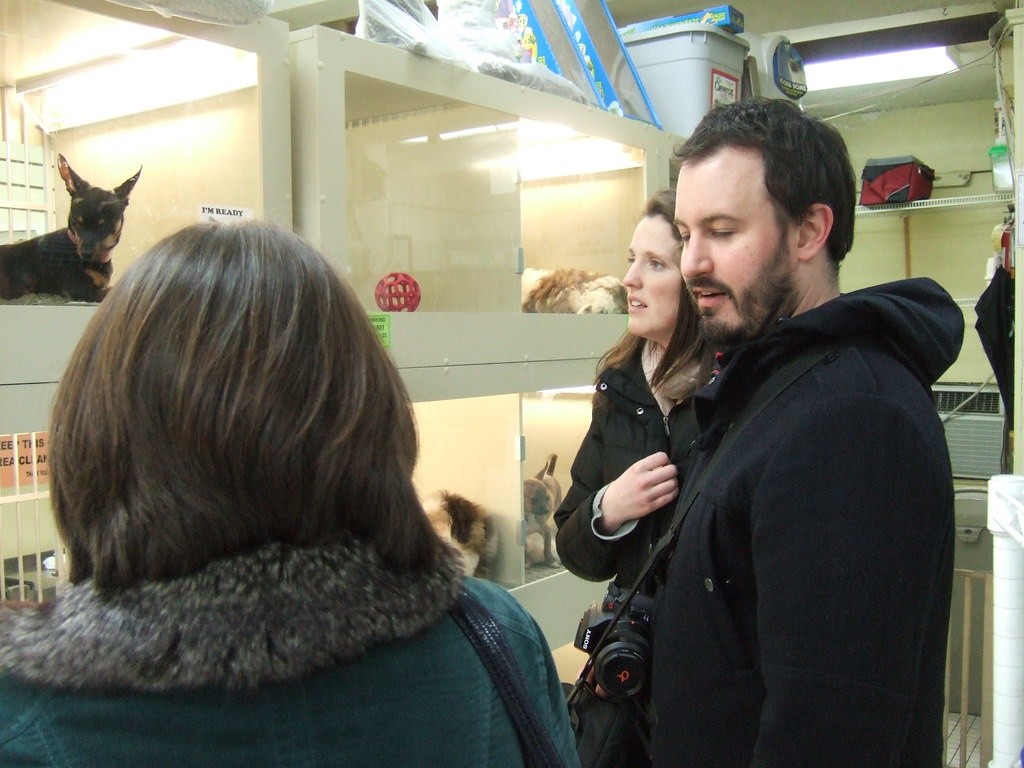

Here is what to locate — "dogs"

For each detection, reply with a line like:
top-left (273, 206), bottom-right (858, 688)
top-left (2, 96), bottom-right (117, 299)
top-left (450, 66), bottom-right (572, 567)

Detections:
top-left (421, 490), bottom-right (499, 578)
top-left (520, 268), bottom-right (627, 314)
top-left (0, 153), bottom-right (143, 305)
top-left (523, 453), bottom-right (562, 570)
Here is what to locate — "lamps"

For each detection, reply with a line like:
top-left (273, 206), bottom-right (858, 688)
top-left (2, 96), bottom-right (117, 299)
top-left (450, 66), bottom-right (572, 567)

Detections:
top-left (802, 45), bottom-right (965, 96)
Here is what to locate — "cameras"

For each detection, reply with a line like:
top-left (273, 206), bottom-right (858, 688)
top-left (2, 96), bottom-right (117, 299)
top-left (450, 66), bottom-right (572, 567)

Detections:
top-left (574, 589), bottom-right (654, 699)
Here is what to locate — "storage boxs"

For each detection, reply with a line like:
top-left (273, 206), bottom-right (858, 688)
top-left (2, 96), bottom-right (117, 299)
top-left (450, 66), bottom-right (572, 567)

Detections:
top-left (619, 17), bottom-right (749, 133)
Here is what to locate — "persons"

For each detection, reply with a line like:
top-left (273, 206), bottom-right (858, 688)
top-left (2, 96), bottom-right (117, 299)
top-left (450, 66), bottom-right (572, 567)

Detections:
top-left (552, 188), bottom-right (729, 583)
top-left (584, 94), bottom-right (968, 767)
top-left (1, 214), bottom-right (583, 768)
